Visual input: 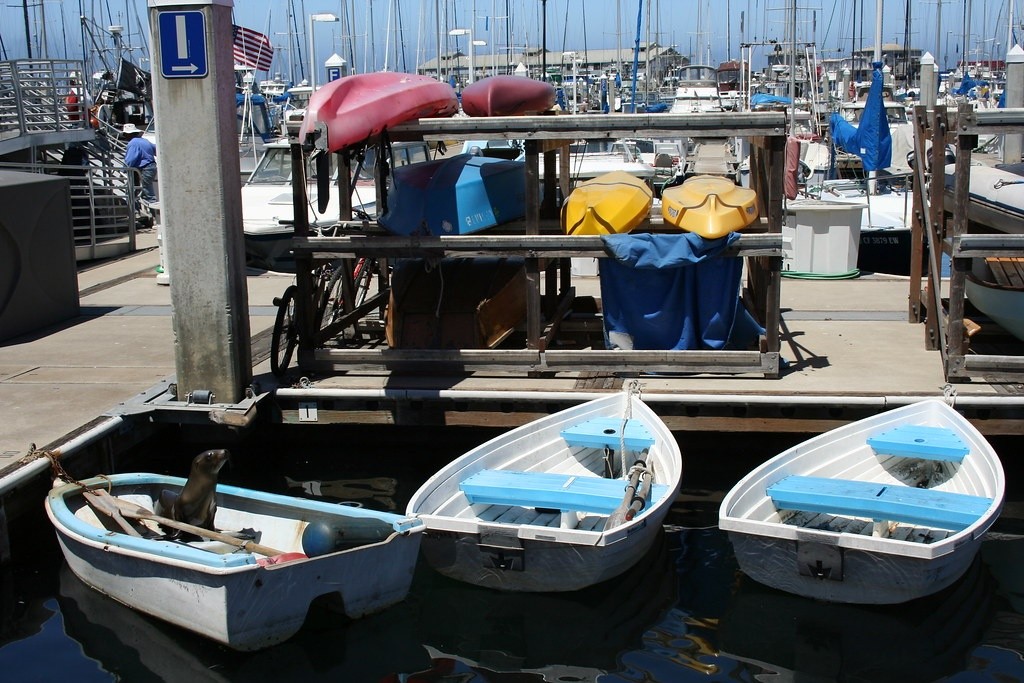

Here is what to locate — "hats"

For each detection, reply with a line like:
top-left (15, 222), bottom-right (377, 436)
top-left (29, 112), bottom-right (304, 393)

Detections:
top-left (119, 124), bottom-right (144, 137)
top-left (69, 71), bottom-right (87, 84)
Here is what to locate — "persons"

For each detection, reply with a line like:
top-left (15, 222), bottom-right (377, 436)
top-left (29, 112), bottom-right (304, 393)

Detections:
top-left (118, 124), bottom-right (157, 226)
top-left (65, 71), bottom-right (95, 175)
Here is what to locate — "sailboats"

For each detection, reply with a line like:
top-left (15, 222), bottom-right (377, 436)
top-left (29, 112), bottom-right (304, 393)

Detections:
top-left (0, 0), bottom-right (1024, 346)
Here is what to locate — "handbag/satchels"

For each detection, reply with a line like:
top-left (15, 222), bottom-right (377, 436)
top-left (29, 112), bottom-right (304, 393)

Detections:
top-left (72, 88), bottom-right (94, 109)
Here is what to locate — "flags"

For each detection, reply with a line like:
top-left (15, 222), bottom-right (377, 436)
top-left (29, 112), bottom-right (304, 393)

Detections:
top-left (232, 24), bottom-right (274, 72)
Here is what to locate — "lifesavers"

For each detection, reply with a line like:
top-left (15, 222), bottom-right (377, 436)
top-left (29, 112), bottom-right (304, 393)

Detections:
top-left (67, 87), bottom-right (92, 124)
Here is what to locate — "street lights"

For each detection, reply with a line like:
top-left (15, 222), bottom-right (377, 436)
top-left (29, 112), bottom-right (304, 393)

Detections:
top-left (467, 39), bottom-right (487, 84)
top-left (308, 12), bottom-right (339, 97)
top-left (561, 51), bottom-right (576, 115)
top-left (447, 28), bottom-right (473, 84)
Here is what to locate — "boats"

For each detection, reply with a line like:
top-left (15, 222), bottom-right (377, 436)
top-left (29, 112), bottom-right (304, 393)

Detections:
top-left (717, 397), bottom-right (1000, 607)
top-left (404, 389), bottom-right (684, 595)
top-left (45, 469), bottom-right (427, 657)
top-left (559, 171), bottom-right (654, 236)
top-left (660, 174), bottom-right (759, 241)
